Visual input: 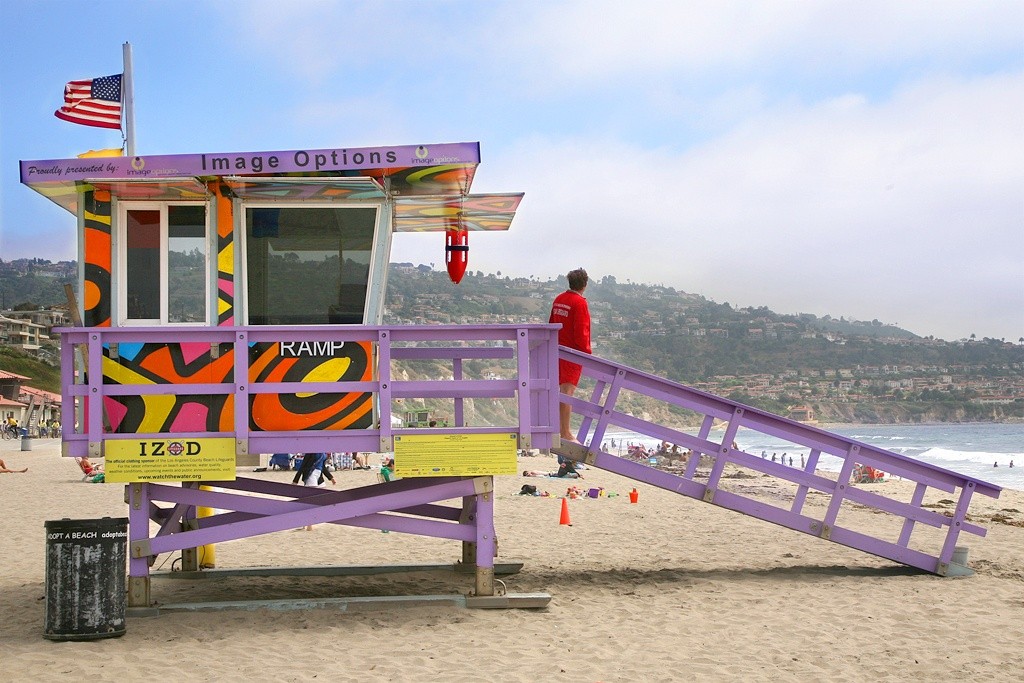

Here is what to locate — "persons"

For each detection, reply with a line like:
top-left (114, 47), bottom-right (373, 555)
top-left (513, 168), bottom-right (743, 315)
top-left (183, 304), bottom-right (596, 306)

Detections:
top-left (993, 459), bottom-right (1014, 469)
top-left (549, 268), bottom-right (592, 446)
top-left (2, 416), bottom-right (79, 439)
top-left (523, 454), bottom-right (584, 480)
top-left (376, 458), bottom-right (397, 483)
top-left (293, 453), bottom-right (336, 530)
top-left (0, 458), bottom-right (28, 473)
top-left (409, 421), bottom-right (437, 428)
top-left (352, 452), bottom-right (370, 469)
top-left (602, 438), bottom-right (807, 483)
top-left (81, 456), bottom-right (105, 476)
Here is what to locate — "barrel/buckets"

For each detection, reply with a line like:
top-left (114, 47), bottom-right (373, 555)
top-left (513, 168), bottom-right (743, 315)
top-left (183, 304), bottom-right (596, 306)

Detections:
top-left (951, 547), bottom-right (968, 563)
top-left (589, 488), bottom-right (599, 498)
top-left (21, 436), bottom-right (32, 451)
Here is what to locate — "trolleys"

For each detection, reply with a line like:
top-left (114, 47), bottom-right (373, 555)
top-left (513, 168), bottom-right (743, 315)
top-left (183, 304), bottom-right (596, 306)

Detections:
top-left (39, 427), bottom-right (49, 439)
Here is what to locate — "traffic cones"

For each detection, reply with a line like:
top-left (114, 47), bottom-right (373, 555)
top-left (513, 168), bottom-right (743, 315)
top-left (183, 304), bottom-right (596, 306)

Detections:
top-left (560, 497), bottom-right (573, 526)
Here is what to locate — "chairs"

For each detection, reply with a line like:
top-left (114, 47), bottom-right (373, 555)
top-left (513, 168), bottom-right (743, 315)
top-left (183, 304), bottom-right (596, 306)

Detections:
top-left (74, 457), bottom-right (103, 482)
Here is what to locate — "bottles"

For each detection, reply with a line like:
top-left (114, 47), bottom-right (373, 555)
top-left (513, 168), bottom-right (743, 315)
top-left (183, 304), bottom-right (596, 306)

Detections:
top-left (630, 488), bottom-right (638, 503)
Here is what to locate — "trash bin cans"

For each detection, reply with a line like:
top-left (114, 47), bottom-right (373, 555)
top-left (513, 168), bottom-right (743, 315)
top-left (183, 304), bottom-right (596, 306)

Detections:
top-left (43, 517), bottom-right (130, 642)
top-left (21, 436), bottom-right (32, 451)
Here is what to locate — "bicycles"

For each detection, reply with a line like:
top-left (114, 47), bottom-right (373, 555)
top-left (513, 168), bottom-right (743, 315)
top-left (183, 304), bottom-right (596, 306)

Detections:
top-left (2, 424), bottom-right (18, 441)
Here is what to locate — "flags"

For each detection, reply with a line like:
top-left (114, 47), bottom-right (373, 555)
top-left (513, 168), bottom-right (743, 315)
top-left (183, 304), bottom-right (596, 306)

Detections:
top-left (54, 74), bottom-right (123, 131)
